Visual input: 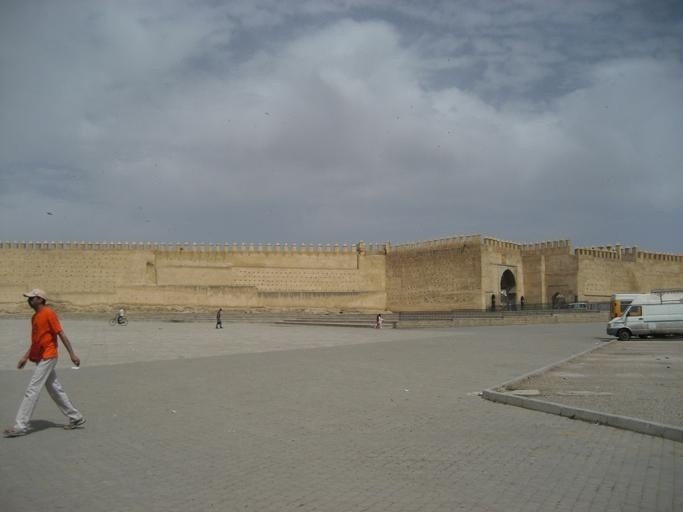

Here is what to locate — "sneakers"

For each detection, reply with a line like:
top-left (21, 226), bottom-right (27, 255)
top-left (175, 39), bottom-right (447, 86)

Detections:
top-left (64, 416), bottom-right (88, 430)
top-left (2, 426), bottom-right (28, 436)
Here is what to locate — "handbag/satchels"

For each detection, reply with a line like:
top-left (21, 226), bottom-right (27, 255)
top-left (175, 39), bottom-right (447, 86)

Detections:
top-left (29, 344), bottom-right (44, 362)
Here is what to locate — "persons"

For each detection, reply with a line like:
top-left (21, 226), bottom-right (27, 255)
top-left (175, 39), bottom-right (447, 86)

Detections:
top-left (115, 307), bottom-right (125, 324)
top-left (215, 307), bottom-right (224, 329)
top-left (0, 287), bottom-right (86, 438)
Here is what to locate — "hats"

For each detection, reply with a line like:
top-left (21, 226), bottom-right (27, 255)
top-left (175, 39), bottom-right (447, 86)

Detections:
top-left (23, 289), bottom-right (48, 300)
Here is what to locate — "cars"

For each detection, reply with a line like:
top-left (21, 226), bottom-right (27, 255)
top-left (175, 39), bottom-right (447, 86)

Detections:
top-left (566, 303), bottom-right (588, 313)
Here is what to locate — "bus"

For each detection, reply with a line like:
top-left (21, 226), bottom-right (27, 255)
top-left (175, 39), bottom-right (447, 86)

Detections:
top-left (607, 291), bottom-right (641, 320)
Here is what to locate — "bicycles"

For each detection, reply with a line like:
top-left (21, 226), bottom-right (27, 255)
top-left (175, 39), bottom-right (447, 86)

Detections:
top-left (109, 312), bottom-right (129, 327)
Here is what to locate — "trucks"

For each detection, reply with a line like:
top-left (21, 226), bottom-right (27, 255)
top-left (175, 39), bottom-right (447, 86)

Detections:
top-left (607, 286), bottom-right (683, 341)
